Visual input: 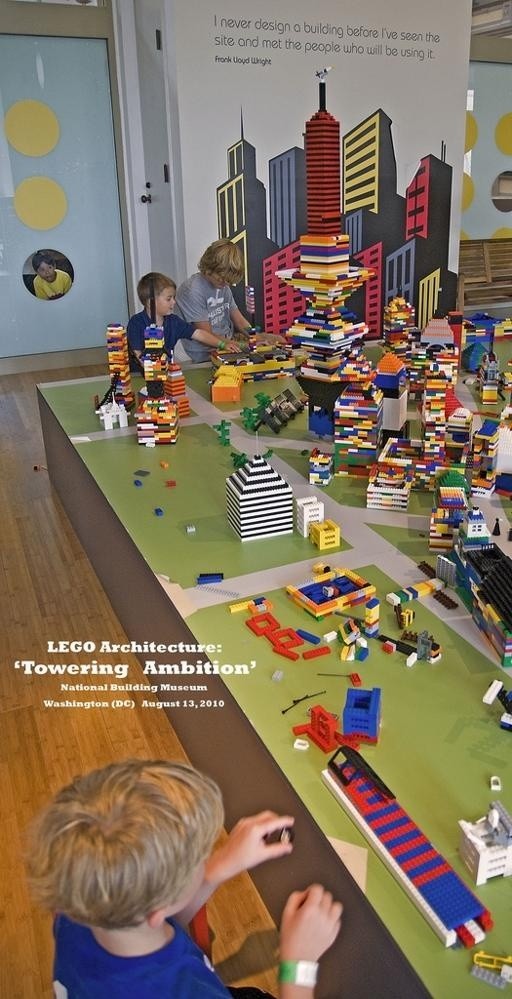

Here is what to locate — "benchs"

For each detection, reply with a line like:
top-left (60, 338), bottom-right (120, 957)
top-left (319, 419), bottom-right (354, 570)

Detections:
top-left (459, 236), bottom-right (512, 313)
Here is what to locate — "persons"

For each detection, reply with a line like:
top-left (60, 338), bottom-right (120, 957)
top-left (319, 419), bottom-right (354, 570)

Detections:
top-left (32, 252), bottom-right (72, 301)
top-left (21, 759), bottom-right (344, 998)
top-left (92, 234), bottom-right (512, 994)
